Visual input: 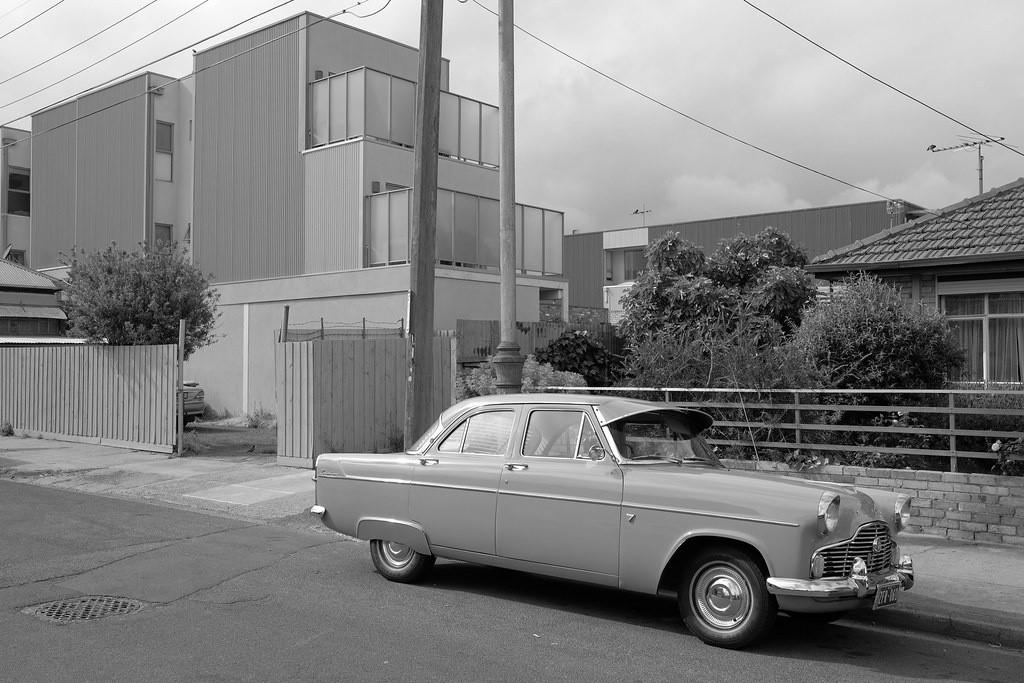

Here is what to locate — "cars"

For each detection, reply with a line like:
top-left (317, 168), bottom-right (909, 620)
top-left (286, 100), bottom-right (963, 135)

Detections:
top-left (309, 393), bottom-right (914, 651)
